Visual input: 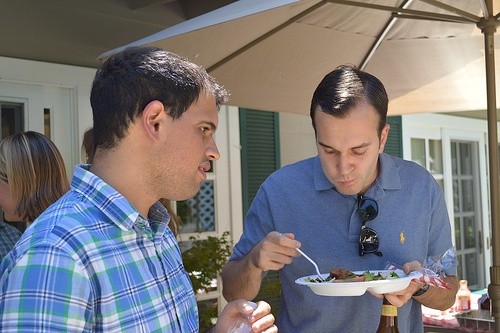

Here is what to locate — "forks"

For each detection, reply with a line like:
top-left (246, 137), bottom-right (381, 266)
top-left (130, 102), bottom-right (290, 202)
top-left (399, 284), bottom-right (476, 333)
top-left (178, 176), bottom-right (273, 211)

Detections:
top-left (280, 234), bottom-right (325, 282)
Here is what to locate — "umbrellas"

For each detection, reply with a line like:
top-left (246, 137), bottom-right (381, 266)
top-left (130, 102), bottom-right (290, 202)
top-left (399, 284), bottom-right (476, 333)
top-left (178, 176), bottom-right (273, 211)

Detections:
top-left (96, 0), bottom-right (500, 319)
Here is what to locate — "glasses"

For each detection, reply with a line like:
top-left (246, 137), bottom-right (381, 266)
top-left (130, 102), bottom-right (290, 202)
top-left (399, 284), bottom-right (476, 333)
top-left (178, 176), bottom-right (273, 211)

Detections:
top-left (357, 194), bottom-right (383, 258)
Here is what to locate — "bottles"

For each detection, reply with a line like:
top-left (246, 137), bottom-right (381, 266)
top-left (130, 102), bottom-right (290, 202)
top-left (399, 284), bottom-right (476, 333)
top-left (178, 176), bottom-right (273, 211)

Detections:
top-left (376, 305), bottom-right (400, 333)
top-left (227, 301), bottom-right (257, 333)
top-left (456, 280), bottom-right (472, 313)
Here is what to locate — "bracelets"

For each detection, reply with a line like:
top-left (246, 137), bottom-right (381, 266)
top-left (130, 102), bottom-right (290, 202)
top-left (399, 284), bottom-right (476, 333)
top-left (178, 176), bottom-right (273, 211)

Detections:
top-left (412, 285), bottom-right (428, 299)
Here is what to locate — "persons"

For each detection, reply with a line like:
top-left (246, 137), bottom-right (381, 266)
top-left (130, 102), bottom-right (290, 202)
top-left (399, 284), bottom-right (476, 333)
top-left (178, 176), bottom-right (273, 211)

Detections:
top-left (0, 45), bottom-right (280, 333)
top-left (0, 130), bottom-right (72, 264)
top-left (82, 127), bottom-right (181, 240)
top-left (221, 64), bottom-right (461, 333)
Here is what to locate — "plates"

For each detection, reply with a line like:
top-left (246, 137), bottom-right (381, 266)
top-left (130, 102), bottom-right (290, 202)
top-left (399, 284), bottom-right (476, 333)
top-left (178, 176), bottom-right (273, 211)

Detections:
top-left (295, 270), bottom-right (423, 297)
